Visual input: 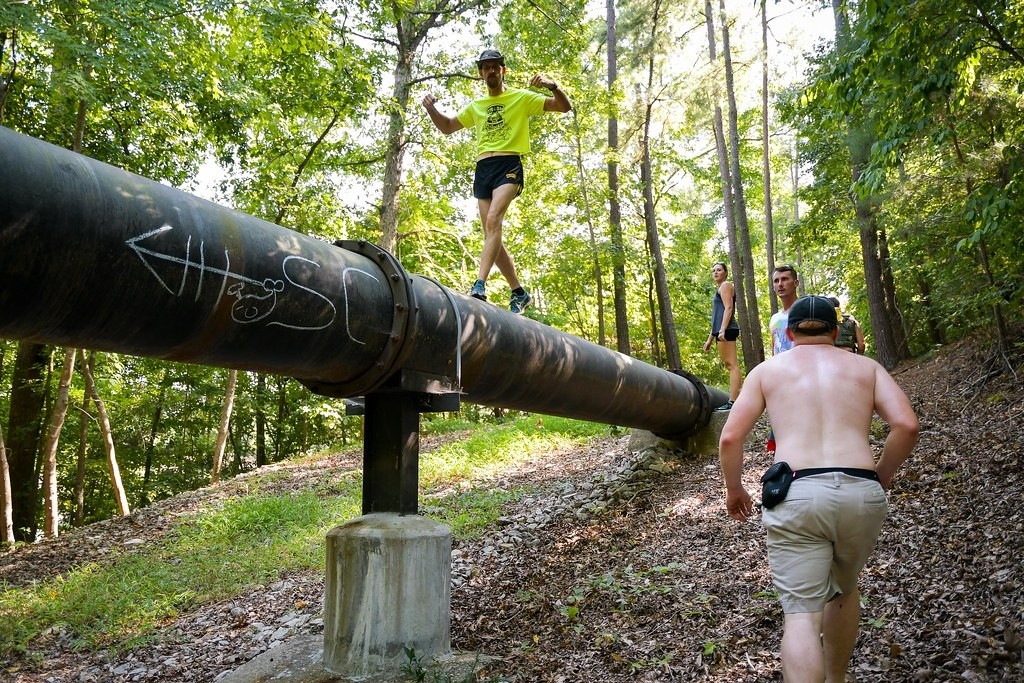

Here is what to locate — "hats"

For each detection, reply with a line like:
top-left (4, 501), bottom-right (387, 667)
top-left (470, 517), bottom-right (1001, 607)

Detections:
top-left (788, 296), bottom-right (837, 333)
top-left (475, 50), bottom-right (505, 63)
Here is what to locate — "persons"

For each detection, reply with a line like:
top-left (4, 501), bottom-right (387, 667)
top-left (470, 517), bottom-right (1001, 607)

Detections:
top-left (421, 49), bottom-right (573, 315)
top-left (702, 262), bottom-right (743, 413)
top-left (715, 292), bottom-right (922, 683)
top-left (827, 295), bottom-right (866, 355)
top-left (766, 264), bottom-right (809, 356)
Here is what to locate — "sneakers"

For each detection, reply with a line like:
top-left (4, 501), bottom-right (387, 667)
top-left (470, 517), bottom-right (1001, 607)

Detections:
top-left (714, 400), bottom-right (736, 413)
top-left (472, 279), bottom-right (487, 301)
top-left (510, 289), bottom-right (533, 315)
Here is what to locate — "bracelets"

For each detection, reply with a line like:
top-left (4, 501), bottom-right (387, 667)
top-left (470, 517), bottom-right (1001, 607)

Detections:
top-left (719, 329), bottom-right (726, 333)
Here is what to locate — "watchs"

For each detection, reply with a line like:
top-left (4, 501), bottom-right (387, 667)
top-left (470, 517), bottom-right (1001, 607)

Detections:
top-left (548, 80), bottom-right (559, 92)
